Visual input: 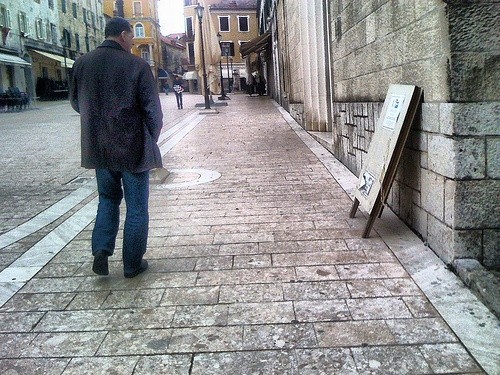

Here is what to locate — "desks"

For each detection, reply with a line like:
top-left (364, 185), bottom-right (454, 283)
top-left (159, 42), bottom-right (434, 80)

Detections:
top-left (0, 92), bottom-right (30, 112)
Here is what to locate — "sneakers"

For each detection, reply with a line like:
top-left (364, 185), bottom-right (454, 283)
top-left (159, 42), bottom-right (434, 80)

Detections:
top-left (122, 259), bottom-right (149, 279)
top-left (92, 248), bottom-right (111, 276)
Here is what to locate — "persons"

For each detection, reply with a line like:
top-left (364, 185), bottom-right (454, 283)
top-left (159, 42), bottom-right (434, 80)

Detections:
top-left (70, 17), bottom-right (163, 278)
top-left (172, 76), bottom-right (184, 110)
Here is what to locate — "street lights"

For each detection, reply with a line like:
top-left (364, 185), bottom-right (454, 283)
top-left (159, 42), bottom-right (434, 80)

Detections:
top-left (194, 3), bottom-right (218, 114)
top-left (229, 57), bottom-right (234, 92)
top-left (60, 37), bottom-right (68, 79)
top-left (216, 32), bottom-right (231, 100)
top-left (225, 47), bottom-right (234, 93)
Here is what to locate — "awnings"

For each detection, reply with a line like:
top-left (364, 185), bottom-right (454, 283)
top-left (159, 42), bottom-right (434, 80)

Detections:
top-left (0, 48), bottom-right (75, 69)
